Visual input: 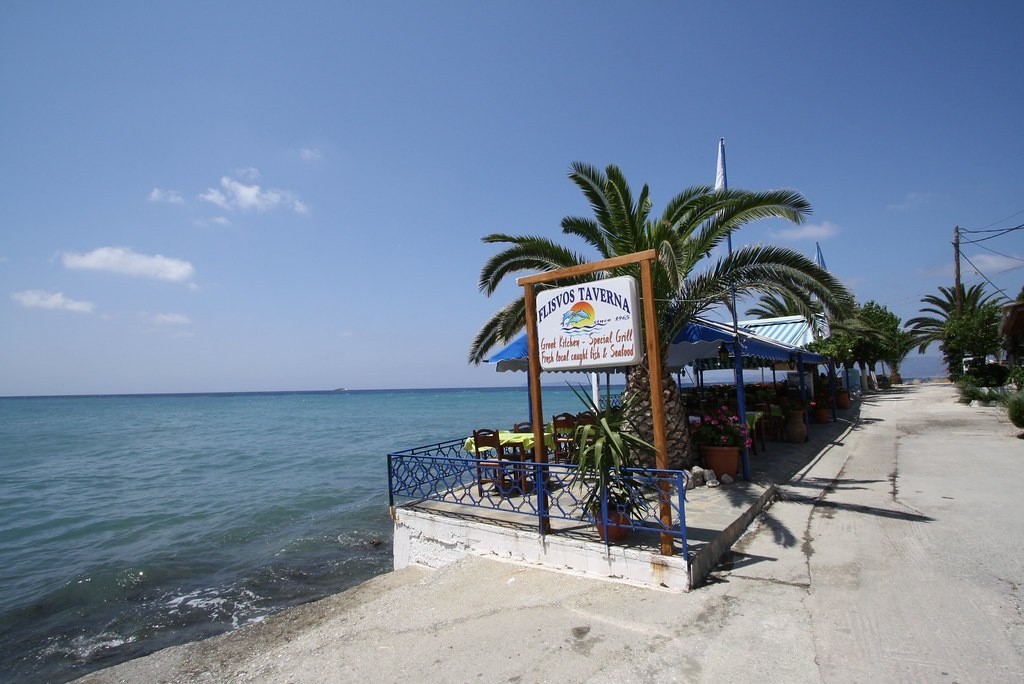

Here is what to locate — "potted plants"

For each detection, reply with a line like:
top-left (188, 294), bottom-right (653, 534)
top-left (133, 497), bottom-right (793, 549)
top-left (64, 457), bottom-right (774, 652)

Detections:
top-left (559, 383), bottom-right (655, 543)
top-left (837, 388), bottom-right (849, 409)
top-left (780, 396), bottom-right (808, 444)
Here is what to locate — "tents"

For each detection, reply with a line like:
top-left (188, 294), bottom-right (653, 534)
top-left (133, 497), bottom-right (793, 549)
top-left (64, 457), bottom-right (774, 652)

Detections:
top-left (483, 306), bottom-right (850, 483)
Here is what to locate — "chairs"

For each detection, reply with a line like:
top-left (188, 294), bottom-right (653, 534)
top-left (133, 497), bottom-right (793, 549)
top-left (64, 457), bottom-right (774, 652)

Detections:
top-left (474, 411), bottom-right (626, 496)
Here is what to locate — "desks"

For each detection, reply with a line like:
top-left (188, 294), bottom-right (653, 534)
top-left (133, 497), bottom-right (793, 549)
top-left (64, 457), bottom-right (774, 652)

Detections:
top-left (466, 431), bottom-right (552, 492)
top-left (745, 411), bottom-right (766, 454)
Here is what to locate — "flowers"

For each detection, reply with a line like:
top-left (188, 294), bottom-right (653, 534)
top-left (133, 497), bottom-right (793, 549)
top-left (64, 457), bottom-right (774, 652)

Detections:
top-left (810, 392), bottom-right (834, 409)
top-left (689, 404), bottom-right (751, 449)
top-left (815, 410), bottom-right (828, 423)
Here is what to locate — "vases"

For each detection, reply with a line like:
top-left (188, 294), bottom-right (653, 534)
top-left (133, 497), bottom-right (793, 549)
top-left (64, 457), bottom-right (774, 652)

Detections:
top-left (703, 447), bottom-right (738, 480)
